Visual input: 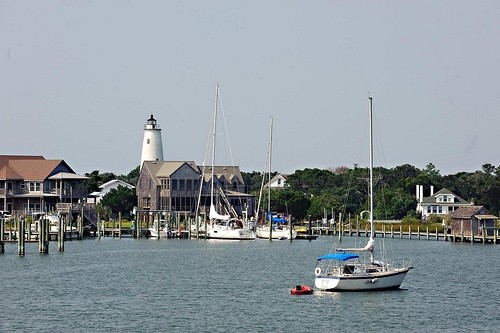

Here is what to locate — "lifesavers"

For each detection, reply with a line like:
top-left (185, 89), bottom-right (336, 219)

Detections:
top-left (315, 268), bottom-right (321, 276)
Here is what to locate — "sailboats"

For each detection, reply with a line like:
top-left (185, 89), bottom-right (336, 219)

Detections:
top-left (315, 97), bottom-right (412, 290)
top-left (190, 84), bottom-right (256, 239)
top-left (252, 114), bottom-right (298, 239)
